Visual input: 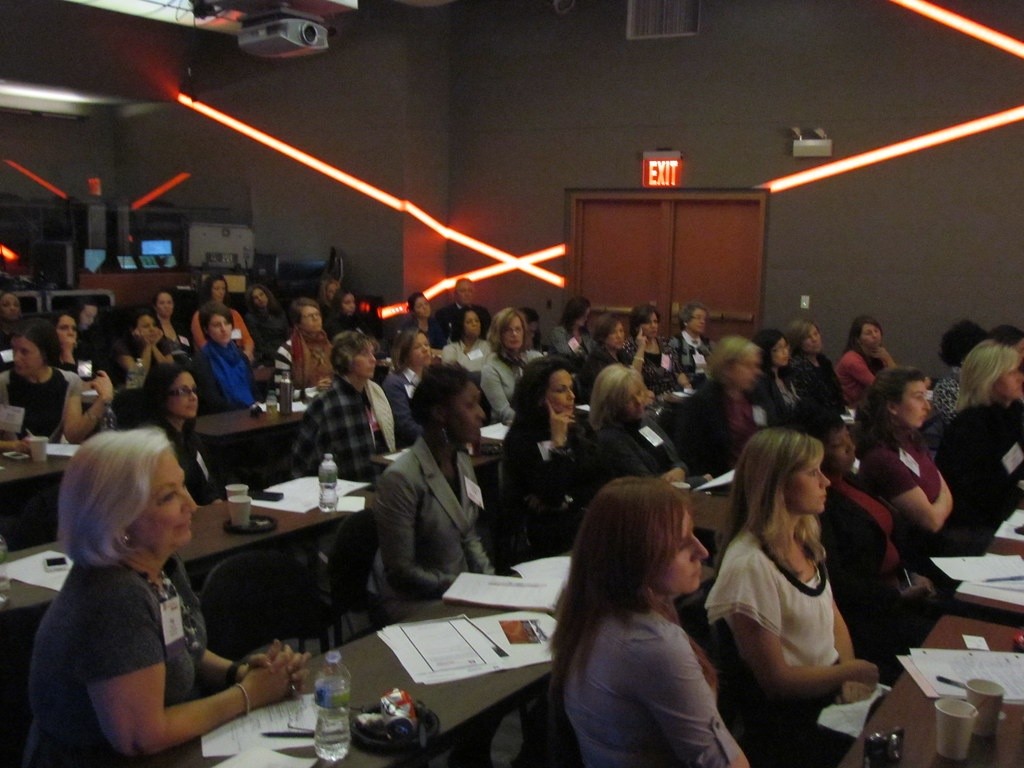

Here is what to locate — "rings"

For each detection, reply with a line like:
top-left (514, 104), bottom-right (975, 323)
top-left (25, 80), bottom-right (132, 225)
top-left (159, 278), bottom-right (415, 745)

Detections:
top-left (289, 683), bottom-right (296, 695)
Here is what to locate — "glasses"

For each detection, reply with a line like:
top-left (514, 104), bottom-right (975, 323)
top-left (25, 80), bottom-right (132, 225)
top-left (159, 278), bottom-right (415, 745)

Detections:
top-left (167, 387), bottom-right (200, 396)
top-left (772, 344), bottom-right (790, 352)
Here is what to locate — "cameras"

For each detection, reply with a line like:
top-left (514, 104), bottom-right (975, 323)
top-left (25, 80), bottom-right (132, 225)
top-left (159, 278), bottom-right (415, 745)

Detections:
top-left (862, 727), bottom-right (904, 768)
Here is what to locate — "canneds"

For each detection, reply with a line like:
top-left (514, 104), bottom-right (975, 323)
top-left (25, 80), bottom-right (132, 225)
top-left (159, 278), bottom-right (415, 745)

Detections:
top-left (379, 688), bottom-right (417, 741)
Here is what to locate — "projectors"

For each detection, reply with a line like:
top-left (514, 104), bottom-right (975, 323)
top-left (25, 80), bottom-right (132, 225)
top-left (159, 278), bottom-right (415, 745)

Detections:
top-left (238, 19), bottom-right (330, 57)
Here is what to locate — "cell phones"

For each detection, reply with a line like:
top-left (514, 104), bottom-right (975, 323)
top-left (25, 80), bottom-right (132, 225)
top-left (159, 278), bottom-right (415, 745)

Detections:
top-left (45, 555), bottom-right (69, 572)
top-left (251, 492), bottom-right (284, 501)
top-left (3, 451), bottom-right (29, 459)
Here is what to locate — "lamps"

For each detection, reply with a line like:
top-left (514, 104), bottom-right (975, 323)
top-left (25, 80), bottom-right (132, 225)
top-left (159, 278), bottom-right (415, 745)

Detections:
top-left (792, 126), bottom-right (833, 156)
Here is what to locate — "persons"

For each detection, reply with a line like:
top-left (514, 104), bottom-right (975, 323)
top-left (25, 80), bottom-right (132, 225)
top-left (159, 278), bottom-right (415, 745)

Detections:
top-left (547, 401), bottom-right (940, 768)
top-left (551, 297), bottom-right (722, 414)
top-left (274, 279), bottom-right (396, 484)
top-left (382, 278), bottom-right (547, 450)
top-left (0, 274), bottom-right (290, 552)
top-left (367, 354), bottom-right (637, 768)
top-left (837, 314), bottom-right (1024, 620)
top-left (684, 317), bottom-right (847, 494)
top-left (23, 425), bottom-right (313, 768)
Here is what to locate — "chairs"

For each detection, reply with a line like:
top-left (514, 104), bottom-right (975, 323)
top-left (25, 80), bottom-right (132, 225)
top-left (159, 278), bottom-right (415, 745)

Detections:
top-left (0, 330), bottom-right (740, 767)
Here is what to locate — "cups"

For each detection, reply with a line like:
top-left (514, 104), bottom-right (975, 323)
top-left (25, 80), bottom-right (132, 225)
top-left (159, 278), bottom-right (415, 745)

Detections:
top-left (965, 677), bottom-right (1006, 736)
top-left (226, 483), bottom-right (249, 499)
top-left (227, 494), bottom-right (251, 529)
top-left (934, 697), bottom-right (978, 760)
top-left (25, 436), bottom-right (49, 462)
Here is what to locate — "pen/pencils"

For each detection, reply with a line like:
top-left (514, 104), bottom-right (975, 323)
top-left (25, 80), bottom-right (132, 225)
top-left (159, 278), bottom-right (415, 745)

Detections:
top-left (936, 675), bottom-right (967, 690)
top-left (261, 731), bottom-right (315, 738)
top-left (983, 575), bottom-right (1024, 583)
top-left (24, 429), bottom-right (34, 437)
top-left (903, 568), bottom-right (912, 587)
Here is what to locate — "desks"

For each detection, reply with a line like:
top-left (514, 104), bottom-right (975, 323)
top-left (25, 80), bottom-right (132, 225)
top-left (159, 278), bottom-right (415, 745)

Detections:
top-left (0, 394), bottom-right (1023, 767)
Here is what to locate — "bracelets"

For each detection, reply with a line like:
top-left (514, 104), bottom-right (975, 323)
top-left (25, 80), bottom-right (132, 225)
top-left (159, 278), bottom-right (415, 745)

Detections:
top-left (225, 661), bottom-right (240, 687)
top-left (634, 356), bottom-right (645, 363)
top-left (234, 683), bottom-right (250, 716)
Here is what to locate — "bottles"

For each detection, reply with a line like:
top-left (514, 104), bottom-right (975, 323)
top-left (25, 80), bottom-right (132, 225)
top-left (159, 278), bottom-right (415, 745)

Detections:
top-left (318, 454), bottom-right (338, 513)
top-left (0, 533), bottom-right (11, 604)
top-left (280, 370), bottom-right (293, 416)
top-left (313, 648), bottom-right (352, 761)
top-left (99, 399), bottom-right (117, 432)
top-left (266, 390), bottom-right (278, 422)
